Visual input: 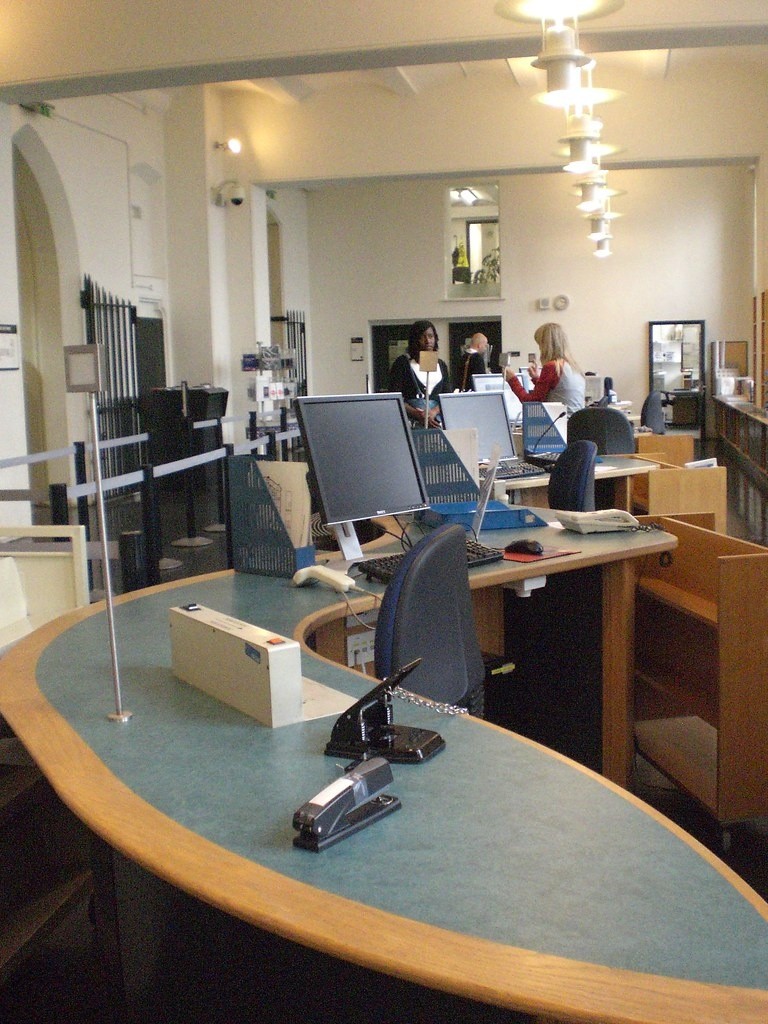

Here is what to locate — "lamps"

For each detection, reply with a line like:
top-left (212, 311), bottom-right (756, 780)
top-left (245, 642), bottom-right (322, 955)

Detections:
top-left (531, 0), bottom-right (617, 255)
top-left (450, 188), bottom-right (478, 206)
top-left (217, 138), bottom-right (240, 153)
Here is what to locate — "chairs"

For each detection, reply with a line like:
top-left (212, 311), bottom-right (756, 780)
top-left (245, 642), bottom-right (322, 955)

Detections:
top-left (640, 390), bottom-right (665, 434)
top-left (548, 440), bottom-right (598, 512)
top-left (373, 524), bottom-right (484, 704)
top-left (567, 407), bottom-right (636, 455)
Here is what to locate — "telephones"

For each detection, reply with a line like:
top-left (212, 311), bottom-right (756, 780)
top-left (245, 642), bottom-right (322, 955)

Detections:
top-left (293, 565), bottom-right (355, 592)
top-left (555, 508), bottom-right (639, 534)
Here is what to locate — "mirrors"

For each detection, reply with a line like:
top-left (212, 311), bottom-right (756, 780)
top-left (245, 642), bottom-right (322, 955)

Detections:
top-left (444, 182), bottom-right (502, 299)
top-left (648, 320), bottom-right (706, 427)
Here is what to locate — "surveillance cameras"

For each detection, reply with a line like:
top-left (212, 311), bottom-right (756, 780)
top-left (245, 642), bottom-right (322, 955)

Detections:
top-left (227, 185), bottom-right (244, 206)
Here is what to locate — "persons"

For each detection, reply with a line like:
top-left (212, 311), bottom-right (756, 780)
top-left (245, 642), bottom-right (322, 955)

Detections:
top-left (454, 333), bottom-right (491, 395)
top-left (390, 320), bottom-right (450, 430)
top-left (502, 323), bottom-right (585, 421)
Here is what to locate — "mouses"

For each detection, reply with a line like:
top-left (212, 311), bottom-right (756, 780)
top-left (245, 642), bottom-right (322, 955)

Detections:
top-left (504, 539), bottom-right (543, 556)
top-left (545, 463), bottom-right (556, 473)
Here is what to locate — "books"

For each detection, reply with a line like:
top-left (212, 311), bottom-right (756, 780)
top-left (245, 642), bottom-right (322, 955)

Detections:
top-left (714, 398), bottom-right (768, 546)
top-left (684, 457), bottom-right (717, 469)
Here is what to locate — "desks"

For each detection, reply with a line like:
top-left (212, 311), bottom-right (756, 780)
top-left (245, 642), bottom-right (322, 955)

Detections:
top-left (0, 504), bottom-right (768, 1024)
top-left (496, 456), bottom-right (659, 515)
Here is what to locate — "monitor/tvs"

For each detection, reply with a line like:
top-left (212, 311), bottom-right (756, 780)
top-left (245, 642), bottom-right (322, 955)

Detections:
top-left (471, 373), bottom-right (524, 426)
top-left (438, 392), bottom-right (520, 465)
top-left (520, 366), bottom-right (543, 393)
top-left (293, 392), bottom-right (430, 561)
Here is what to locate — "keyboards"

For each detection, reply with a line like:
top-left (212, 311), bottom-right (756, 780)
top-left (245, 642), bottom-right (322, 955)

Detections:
top-left (358, 538), bottom-right (505, 586)
top-left (478, 461), bottom-right (547, 480)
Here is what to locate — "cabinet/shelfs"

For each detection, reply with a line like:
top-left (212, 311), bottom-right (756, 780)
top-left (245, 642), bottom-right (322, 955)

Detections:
top-left (632, 512), bottom-right (768, 851)
top-left (0, 524), bottom-right (126, 1024)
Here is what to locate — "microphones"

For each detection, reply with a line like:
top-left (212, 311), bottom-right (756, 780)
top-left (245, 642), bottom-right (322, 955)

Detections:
top-left (525, 411), bottom-right (567, 468)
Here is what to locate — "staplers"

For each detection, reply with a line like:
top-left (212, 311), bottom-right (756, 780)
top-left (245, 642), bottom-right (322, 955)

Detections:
top-left (292, 758), bottom-right (402, 854)
top-left (321, 658), bottom-right (446, 765)
